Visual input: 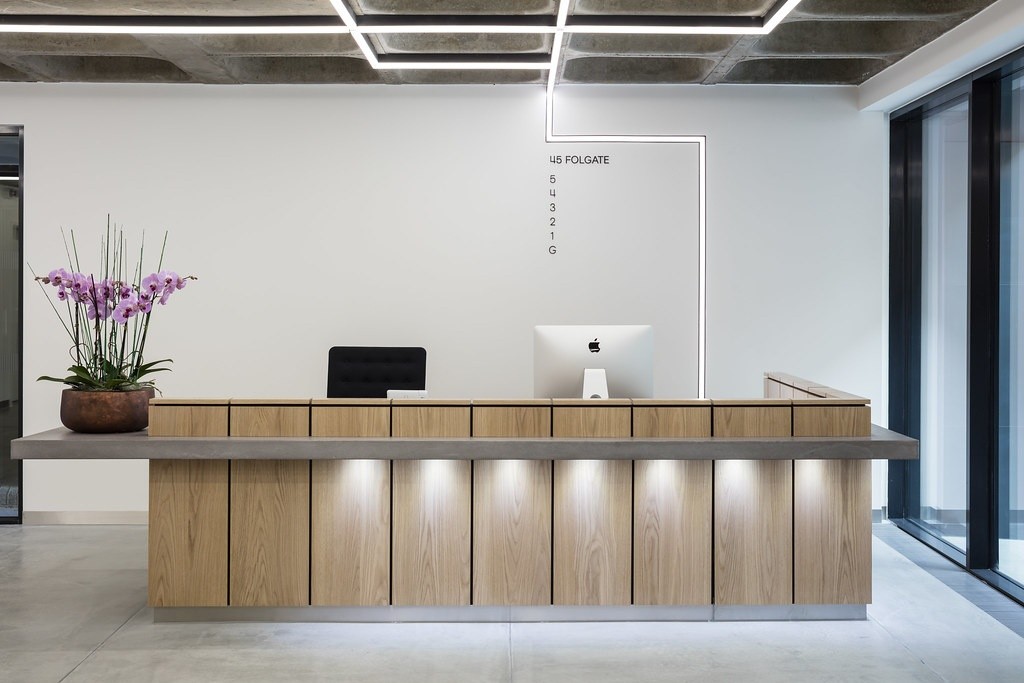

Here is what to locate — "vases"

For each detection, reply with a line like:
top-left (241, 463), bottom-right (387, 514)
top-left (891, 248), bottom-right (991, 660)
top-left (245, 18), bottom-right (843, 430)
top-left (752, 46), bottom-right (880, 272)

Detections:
top-left (59, 388), bottom-right (155, 432)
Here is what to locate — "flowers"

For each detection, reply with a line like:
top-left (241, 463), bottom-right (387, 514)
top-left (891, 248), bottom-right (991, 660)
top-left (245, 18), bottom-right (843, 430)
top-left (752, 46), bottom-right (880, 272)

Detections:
top-left (26, 213), bottom-right (197, 397)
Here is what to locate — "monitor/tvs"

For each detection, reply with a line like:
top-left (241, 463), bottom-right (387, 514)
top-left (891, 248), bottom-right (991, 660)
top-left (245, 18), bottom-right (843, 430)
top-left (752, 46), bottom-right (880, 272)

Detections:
top-left (531, 325), bottom-right (653, 399)
